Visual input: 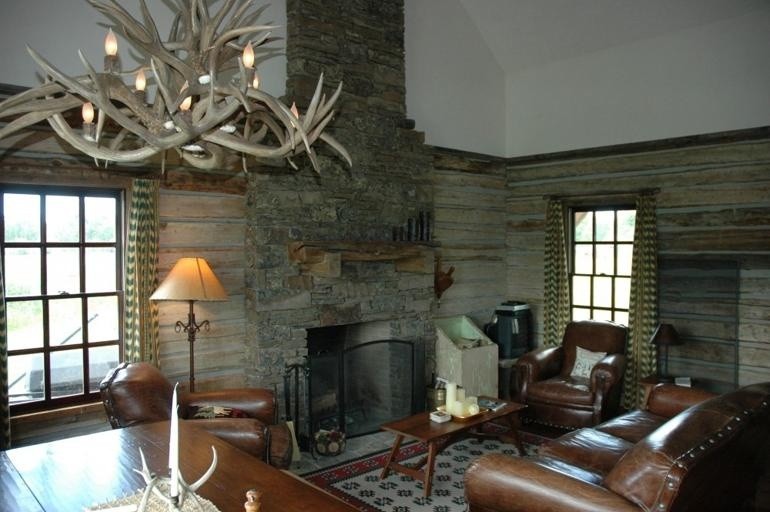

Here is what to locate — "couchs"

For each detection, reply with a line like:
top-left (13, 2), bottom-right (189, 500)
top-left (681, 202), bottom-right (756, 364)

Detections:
top-left (462, 382), bottom-right (770, 512)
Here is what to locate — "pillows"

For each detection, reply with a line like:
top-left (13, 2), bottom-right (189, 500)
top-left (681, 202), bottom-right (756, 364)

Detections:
top-left (570, 345), bottom-right (607, 379)
top-left (194, 407), bottom-right (250, 419)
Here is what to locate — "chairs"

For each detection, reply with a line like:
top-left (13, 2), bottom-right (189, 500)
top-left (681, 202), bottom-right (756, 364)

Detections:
top-left (99, 361), bottom-right (293, 468)
top-left (509, 320), bottom-right (629, 434)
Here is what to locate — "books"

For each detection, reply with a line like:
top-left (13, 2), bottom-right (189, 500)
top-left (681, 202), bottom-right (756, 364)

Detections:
top-left (477, 399), bottom-right (508, 413)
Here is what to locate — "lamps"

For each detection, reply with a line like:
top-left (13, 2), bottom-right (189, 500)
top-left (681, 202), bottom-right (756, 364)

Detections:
top-left (651, 324), bottom-right (684, 379)
top-left (0, 0), bottom-right (354, 175)
top-left (148, 258), bottom-right (229, 392)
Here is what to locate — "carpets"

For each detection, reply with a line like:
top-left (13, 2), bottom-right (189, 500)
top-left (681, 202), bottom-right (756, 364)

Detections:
top-left (299, 420), bottom-right (554, 511)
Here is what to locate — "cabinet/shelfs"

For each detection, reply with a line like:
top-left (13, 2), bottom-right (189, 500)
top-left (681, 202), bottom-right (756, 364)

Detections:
top-left (433, 315), bottom-right (501, 401)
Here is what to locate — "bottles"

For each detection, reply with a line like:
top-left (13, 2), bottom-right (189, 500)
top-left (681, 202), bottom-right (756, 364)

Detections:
top-left (243, 488), bottom-right (263, 512)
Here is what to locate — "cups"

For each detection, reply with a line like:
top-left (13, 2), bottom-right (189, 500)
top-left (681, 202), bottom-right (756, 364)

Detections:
top-left (392, 209), bottom-right (430, 242)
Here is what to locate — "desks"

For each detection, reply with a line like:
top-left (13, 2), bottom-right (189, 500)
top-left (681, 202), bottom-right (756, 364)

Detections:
top-left (0, 418), bottom-right (368, 512)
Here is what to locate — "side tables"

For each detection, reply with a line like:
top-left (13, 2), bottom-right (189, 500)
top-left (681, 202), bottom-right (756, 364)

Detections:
top-left (499, 358), bottom-right (519, 401)
top-left (638, 375), bottom-right (697, 410)
